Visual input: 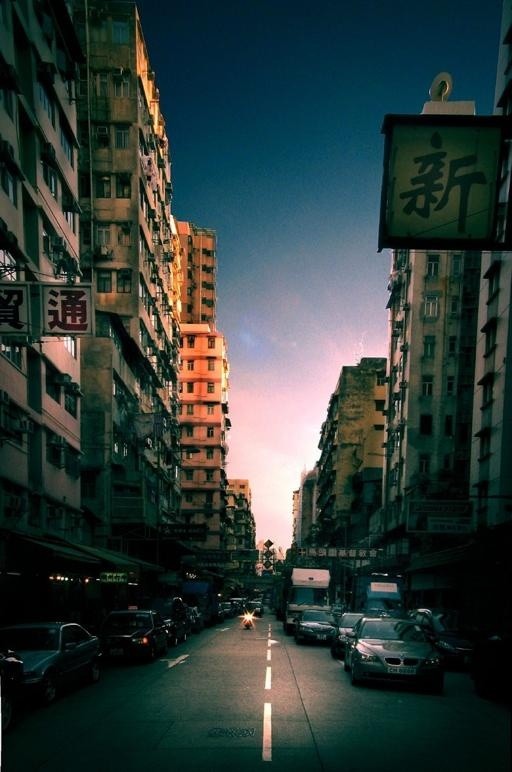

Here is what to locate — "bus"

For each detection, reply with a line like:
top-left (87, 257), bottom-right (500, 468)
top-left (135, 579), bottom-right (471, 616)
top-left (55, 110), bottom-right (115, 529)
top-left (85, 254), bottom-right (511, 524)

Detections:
top-left (353, 572), bottom-right (404, 614)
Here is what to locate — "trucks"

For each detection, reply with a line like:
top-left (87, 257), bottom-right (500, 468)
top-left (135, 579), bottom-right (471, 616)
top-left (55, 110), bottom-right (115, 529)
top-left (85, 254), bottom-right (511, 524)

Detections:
top-left (277, 567), bottom-right (331, 635)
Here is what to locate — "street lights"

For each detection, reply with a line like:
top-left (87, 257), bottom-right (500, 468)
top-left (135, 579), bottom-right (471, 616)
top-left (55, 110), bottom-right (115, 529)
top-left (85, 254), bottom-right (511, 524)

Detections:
top-left (323, 517), bottom-right (347, 603)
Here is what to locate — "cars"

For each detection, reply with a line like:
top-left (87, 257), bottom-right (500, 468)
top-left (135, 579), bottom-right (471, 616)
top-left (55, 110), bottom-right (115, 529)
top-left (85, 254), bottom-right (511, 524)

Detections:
top-left (293, 609), bottom-right (512, 701)
